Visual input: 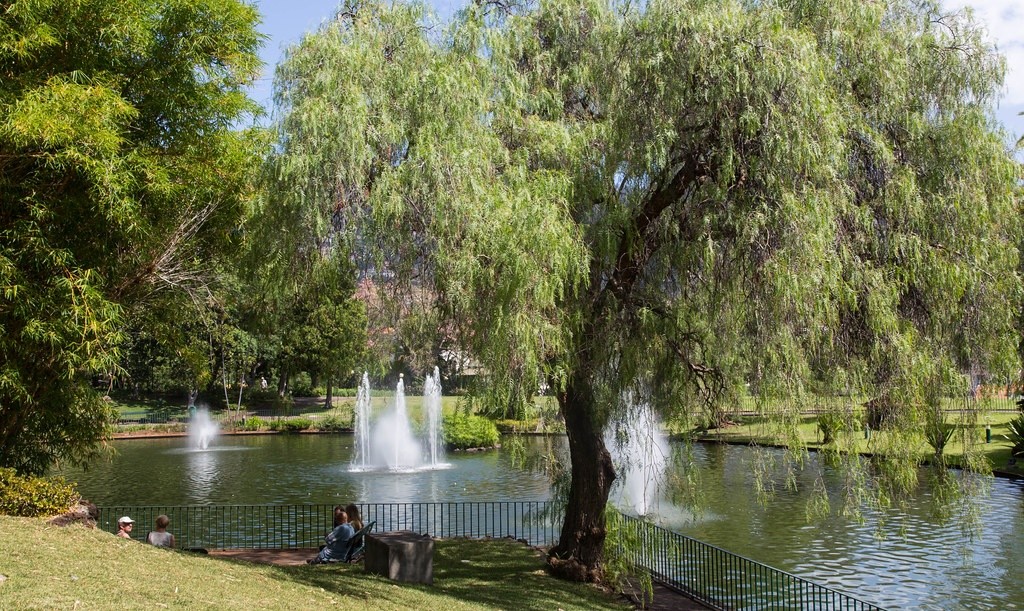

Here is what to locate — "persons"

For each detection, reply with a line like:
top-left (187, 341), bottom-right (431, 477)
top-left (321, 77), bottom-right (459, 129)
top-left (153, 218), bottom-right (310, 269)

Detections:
top-left (307, 503), bottom-right (363, 565)
top-left (116, 516), bottom-right (135, 539)
top-left (146, 515), bottom-right (175, 548)
top-left (261, 377), bottom-right (268, 388)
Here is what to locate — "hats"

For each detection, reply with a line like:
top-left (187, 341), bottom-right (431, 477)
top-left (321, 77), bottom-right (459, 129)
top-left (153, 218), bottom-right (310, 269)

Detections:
top-left (118, 516), bottom-right (135, 523)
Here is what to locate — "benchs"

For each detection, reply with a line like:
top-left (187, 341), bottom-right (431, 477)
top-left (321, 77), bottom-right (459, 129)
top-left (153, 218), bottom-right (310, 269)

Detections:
top-left (322, 521), bottom-right (376, 565)
